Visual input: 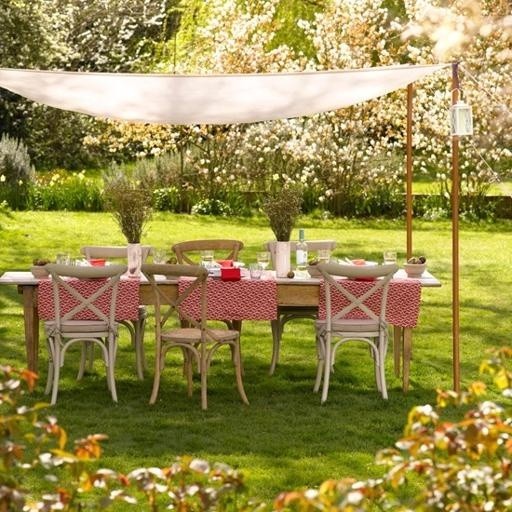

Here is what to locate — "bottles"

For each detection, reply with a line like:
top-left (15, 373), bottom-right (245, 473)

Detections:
top-left (295, 230), bottom-right (309, 280)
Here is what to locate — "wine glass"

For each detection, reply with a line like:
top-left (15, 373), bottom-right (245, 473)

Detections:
top-left (257, 251), bottom-right (269, 275)
top-left (153, 248), bottom-right (166, 277)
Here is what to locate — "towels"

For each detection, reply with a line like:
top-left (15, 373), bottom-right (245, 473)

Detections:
top-left (318, 279), bottom-right (422, 330)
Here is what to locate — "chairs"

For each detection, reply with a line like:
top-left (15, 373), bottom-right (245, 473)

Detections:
top-left (76, 244), bottom-right (151, 383)
top-left (261, 241), bottom-right (337, 378)
top-left (43, 260), bottom-right (130, 404)
top-left (313, 261), bottom-right (398, 404)
top-left (172, 239), bottom-right (247, 379)
top-left (139, 262), bottom-right (251, 409)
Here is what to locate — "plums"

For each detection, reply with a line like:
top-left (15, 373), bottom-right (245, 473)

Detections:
top-left (287, 271), bottom-right (295, 278)
top-left (419, 256), bottom-right (426, 264)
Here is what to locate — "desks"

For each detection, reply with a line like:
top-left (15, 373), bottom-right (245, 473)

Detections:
top-left (0, 260), bottom-right (442, 396)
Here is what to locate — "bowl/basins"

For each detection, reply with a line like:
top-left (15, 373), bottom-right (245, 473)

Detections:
top-left (402, 263), bottom-right (426, 278)
top-left (30, 266), bottom-right (50, 279)
top-left (307, 265), bottom-right (323, 278)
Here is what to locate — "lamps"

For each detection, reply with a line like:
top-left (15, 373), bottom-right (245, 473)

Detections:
top-left (448, 88), bottom-right (474, 137)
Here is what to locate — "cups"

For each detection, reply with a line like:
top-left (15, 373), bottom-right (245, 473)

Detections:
top-left (249, 263), bottom-right (262, 279)
top-left (384, 250), bottom-right (397, 265)
